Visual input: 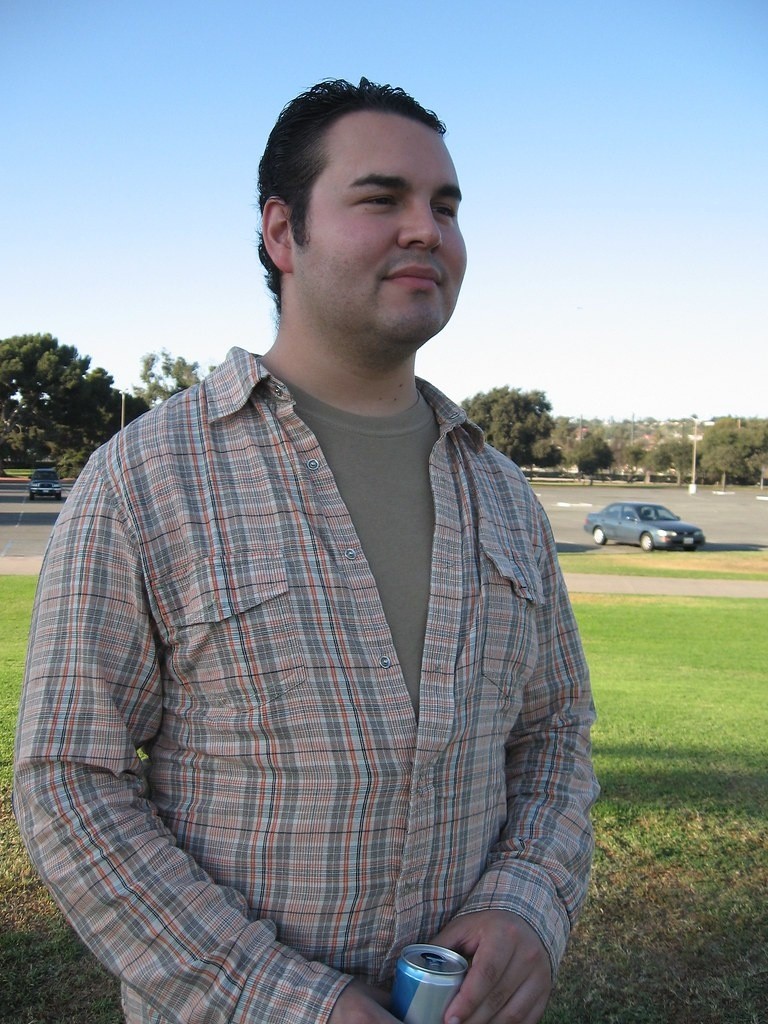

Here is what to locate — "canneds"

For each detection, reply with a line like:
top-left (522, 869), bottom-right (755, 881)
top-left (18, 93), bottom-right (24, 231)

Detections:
top-left (389, 944), bottom-right (468, 1024)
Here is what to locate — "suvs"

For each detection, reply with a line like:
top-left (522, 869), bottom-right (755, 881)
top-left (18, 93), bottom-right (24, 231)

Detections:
top-left (28, 468), bottom-right (66, 501)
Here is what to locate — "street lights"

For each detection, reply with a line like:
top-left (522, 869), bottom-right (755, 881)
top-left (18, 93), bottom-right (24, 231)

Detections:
top-left (688, 414), bottom-right (700, 494)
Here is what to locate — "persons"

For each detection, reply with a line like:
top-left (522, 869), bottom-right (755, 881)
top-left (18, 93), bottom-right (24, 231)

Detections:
top-left (14, 75), bottom-right (601, 1024)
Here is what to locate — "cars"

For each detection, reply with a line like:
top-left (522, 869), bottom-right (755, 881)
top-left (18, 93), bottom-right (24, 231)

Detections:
top-left (583, 501), bottom-right (706, 552)
